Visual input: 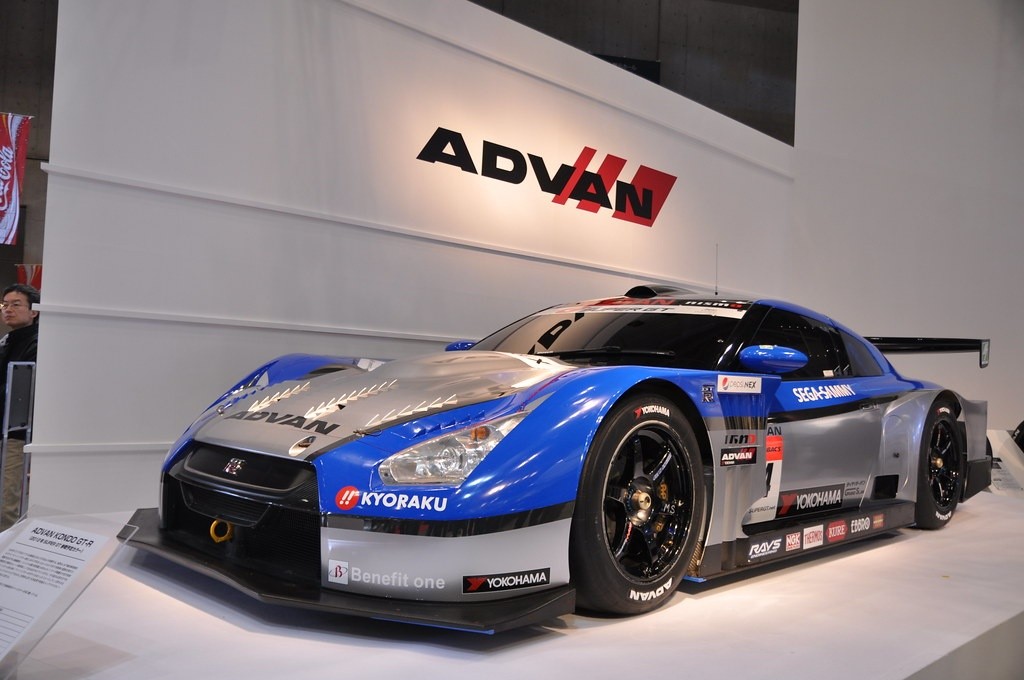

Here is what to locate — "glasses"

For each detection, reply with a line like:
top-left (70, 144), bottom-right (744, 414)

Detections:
top-left (0, 301), bottom-right (31, 312)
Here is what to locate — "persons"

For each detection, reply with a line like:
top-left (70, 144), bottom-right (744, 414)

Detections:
top-left (0, 284), bottom-right (40, 532)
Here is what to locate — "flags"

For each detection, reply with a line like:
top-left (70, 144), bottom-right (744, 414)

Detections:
top-left (0, 113), bottom-right (29, 245)
top-left (17, 266), bottom-right (42, 290)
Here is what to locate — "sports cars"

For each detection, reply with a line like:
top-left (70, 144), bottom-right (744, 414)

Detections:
top-left (116, 284), bottom-right (1003, 635)
